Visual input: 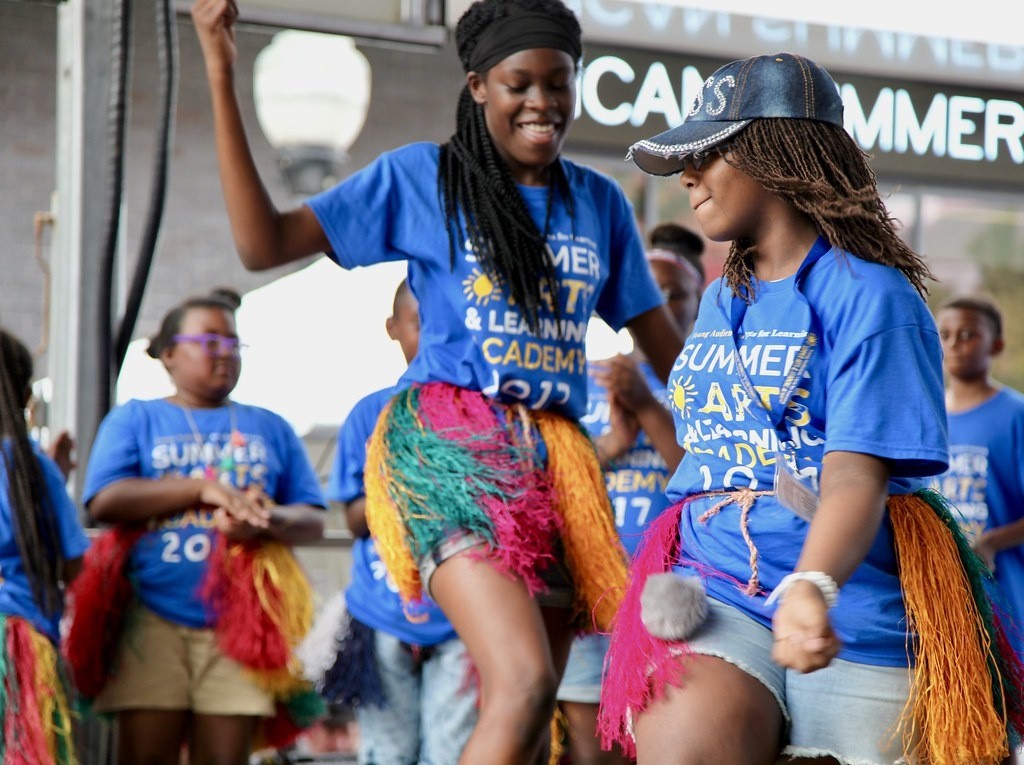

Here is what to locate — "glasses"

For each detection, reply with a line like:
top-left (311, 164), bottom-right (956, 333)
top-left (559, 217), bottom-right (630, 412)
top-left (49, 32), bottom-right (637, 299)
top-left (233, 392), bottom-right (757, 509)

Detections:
top-left (170, 333), bottom-right (247, 359)
top-left (682, 138), bottom-right (771, 171)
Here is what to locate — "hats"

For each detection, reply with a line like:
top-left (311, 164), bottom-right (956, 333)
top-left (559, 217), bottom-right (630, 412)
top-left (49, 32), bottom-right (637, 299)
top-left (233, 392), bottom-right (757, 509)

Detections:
top-left (625, 53), bottom-right (843, 177)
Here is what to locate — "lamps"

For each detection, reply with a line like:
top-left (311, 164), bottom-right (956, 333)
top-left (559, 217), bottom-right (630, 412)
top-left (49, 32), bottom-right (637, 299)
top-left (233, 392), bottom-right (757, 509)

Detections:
top-left (253, 26), bottom-right (372, 197)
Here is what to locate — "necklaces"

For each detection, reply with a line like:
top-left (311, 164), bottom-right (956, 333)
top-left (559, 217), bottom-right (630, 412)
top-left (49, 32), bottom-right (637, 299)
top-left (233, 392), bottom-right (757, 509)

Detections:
top-left (172, 395), bottom-right (237, 482)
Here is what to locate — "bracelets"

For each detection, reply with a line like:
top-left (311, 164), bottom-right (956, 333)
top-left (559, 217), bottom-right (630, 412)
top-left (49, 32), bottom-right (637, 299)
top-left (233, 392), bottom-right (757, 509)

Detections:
top-left (763, 571), bottom-right (839, 608)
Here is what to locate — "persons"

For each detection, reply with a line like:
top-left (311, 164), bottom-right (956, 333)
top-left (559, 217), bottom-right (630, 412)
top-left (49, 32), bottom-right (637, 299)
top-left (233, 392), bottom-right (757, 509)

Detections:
top-left (190, 0), bottom-right (705, 765)
top-left (594, 54), bottom-right (1024, 765)
top-left (80, 289), bottom-right (328, 765)
top-left (0, 327), bottom-right (93, 765)
